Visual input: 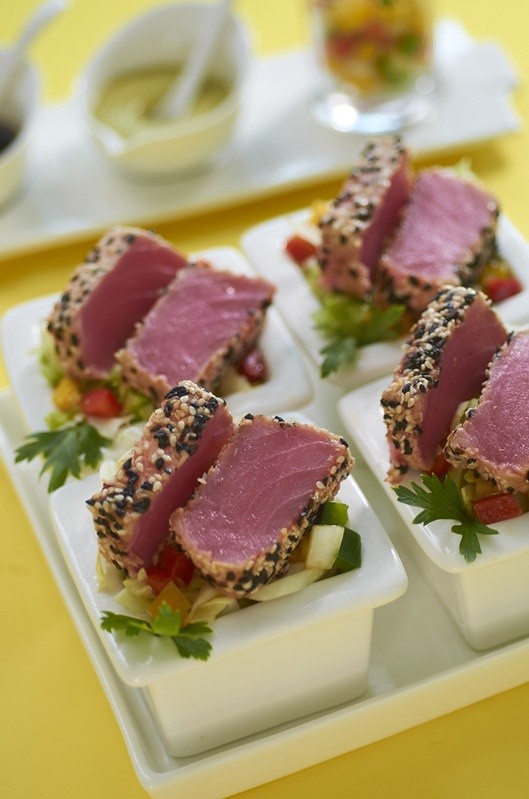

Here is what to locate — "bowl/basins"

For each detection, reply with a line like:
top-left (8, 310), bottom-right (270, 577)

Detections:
top-left (4, 247), bottom-right (313, 470)
top-left (42, 410), bottom-right (416, 758)
top-left (334, 367), bottom-right (529, 653)
top-left (77, 2), bottom-right (251, 180)
top-left (1, 47), bottom-right (52, 212)
top-left (238, 202), bottom-right (529, 404)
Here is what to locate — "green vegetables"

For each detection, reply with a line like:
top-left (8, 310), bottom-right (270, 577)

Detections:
top-left (15, 295), bottom-right (499, 659)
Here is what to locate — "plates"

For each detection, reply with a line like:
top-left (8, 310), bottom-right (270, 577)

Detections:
top-left (1, 17), bottom-right (521, 264)
top-left (3, 376), bottom-right (527, 797)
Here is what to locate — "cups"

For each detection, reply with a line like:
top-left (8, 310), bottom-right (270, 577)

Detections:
top-left (308, 2), bottom-right (438, 136)
